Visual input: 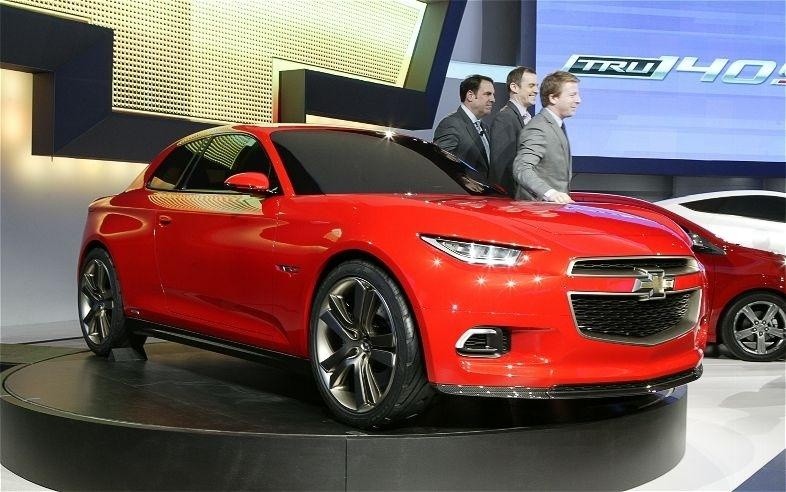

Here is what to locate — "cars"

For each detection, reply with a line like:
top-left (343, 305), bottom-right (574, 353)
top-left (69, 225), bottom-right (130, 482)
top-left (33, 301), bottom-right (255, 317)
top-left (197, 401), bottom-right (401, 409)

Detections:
top-left (77, 122), bottom-right (711, 433)
top-left (571, 190), bottom-right (786, 363)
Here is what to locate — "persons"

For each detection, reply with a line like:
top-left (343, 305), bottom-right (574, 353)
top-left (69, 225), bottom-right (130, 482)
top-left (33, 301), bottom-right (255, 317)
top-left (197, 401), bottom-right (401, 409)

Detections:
top-left (511, 70), bottom-right (582, 205)
top-left (431, 73), bottom-right (495, 180)
top-left (486, 65), bottom-right (541, 194)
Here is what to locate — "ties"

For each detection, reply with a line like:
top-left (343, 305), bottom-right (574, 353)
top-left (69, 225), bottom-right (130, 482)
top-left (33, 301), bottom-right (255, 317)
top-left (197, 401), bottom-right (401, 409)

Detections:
top-left (562, 122), bottom-right (570, 163)
top-left (476, 122), bottom-right (490, 162)
